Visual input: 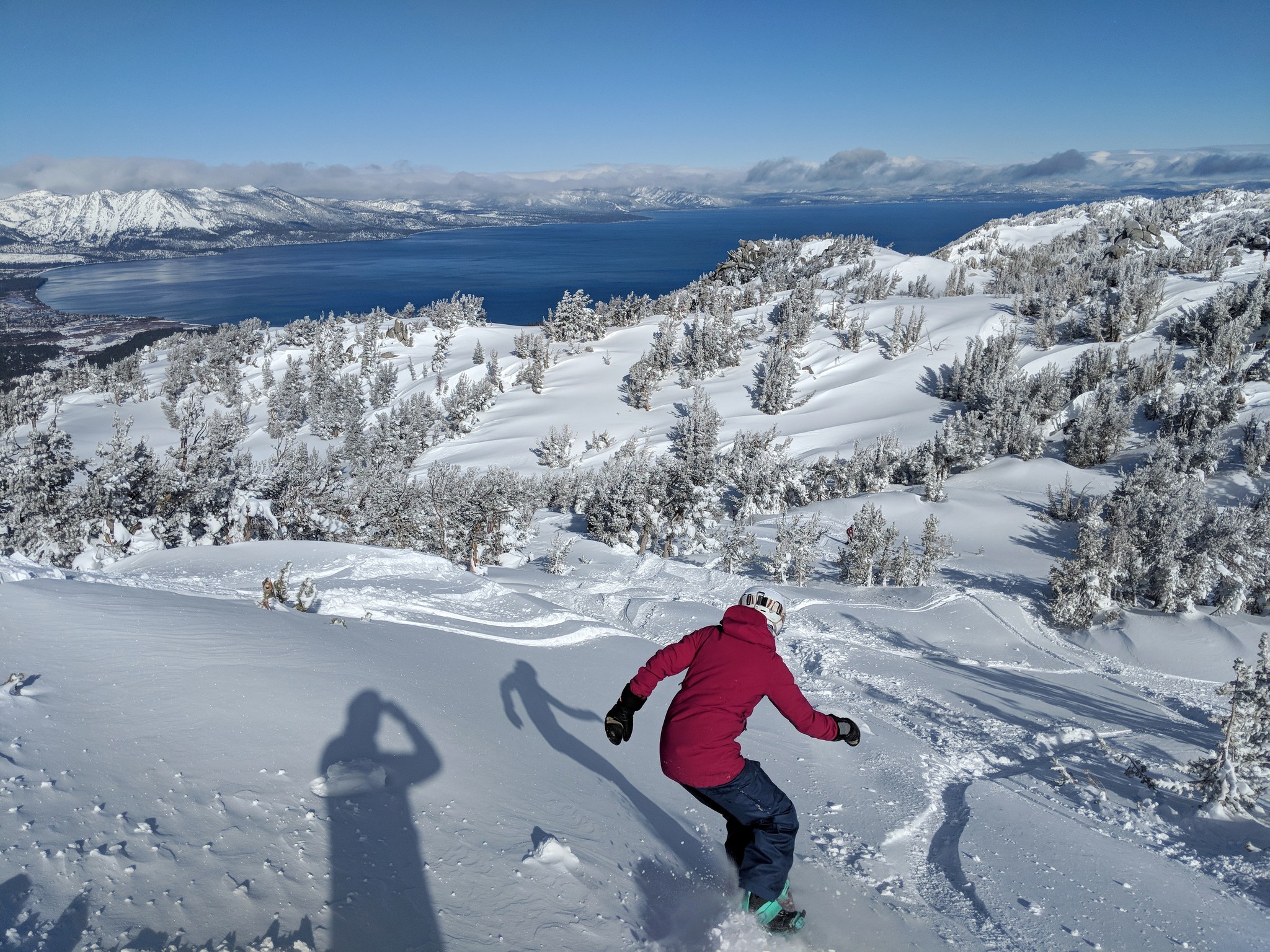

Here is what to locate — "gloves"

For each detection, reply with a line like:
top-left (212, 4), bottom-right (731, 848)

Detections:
top-left (827, 713), bottom-right (861, 747)
top-left (605, 683), bottom-right (647, 746)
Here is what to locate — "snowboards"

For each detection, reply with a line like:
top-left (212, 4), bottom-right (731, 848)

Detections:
top-left (745, 850), bottom-right (803, 938)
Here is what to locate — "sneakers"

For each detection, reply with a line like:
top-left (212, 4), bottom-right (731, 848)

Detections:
top-left (747, 894), bottom-right (799, 933)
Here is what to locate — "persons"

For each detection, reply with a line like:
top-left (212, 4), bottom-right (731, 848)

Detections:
top-left (605, 585), bottom-right (860, 935)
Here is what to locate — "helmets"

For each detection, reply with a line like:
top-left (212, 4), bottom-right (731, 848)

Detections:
top-left (739, 585), bottom-right (786, 637)
